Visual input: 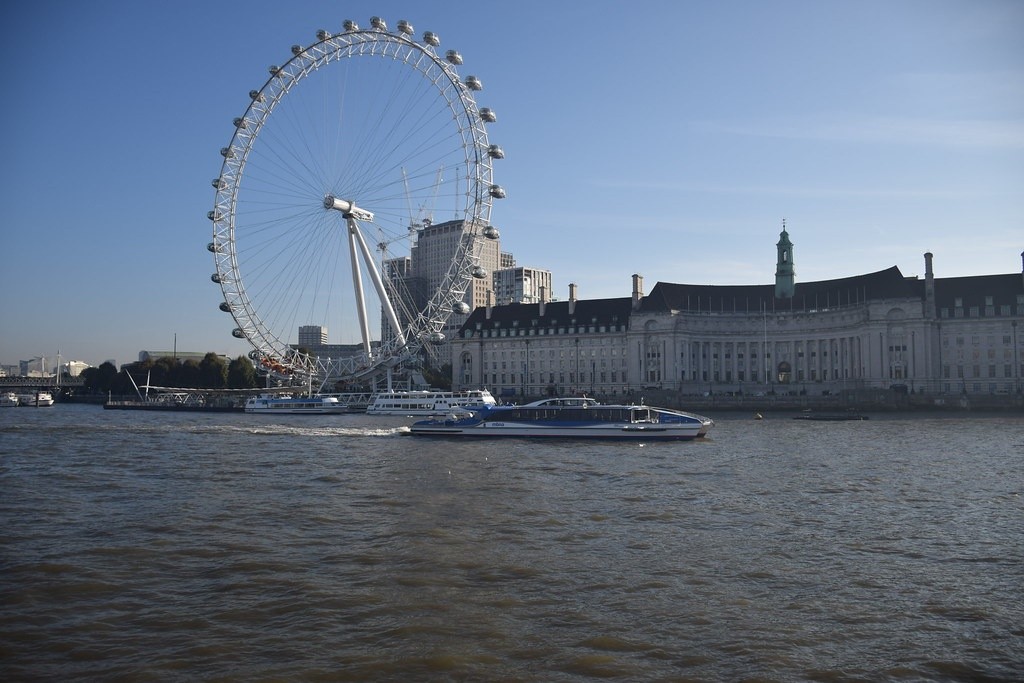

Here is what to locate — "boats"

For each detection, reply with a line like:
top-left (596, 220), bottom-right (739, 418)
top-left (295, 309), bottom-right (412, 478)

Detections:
top-left (244, 394), bottom-right (351, 414)
top-left (0, 391), bottom-right (56, 407)
top-left (366, 386), bottom-right (497, 416)
top-left (410, 397), bottom-right (715, 439)
